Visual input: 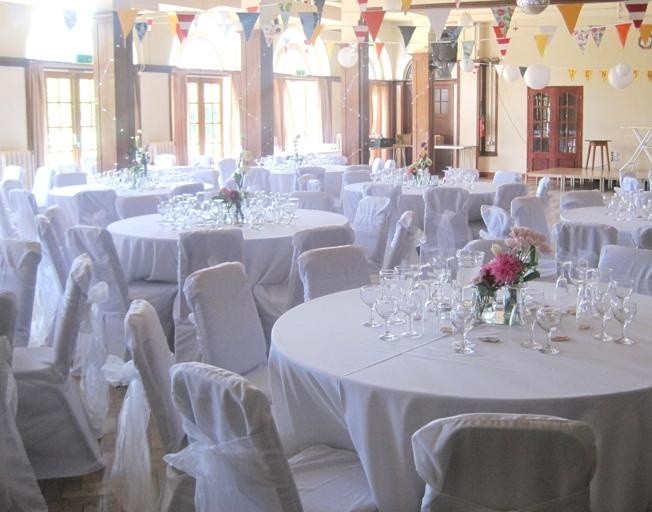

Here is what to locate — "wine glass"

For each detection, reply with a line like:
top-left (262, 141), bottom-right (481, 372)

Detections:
top-left (157, 192), bottom-right (300, 230)
top-left (254, 153), bottom-right (338, 171)
top-left (370, 166), bottom-right (479, 191)
top-left (90, 167), bottom-right (160, 193)
top-left (360, 249), bottom-right (637, 356)
top-left (601, 191), bottom-right (652, 221)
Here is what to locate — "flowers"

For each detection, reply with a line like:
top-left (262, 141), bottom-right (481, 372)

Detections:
top-left (500, 224), bottom-right (554, 287)
top-left (408, 142), bottom-right (433, 176)
top-left (472, 254), bottom-right (523, 309)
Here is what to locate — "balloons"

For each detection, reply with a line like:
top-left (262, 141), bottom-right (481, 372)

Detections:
top-left (522, 64), bottom-right (551, 91)
top-left (458, 12), bottom-right (473, 28)
top-left (615, 62), bottom-right (632, 76)
top-left (460, 59), bottom-right (475, 74)
top-left (501, 64), bottom-right (519, 82)
top-left (520, 0), bottom-right (553, 16)
top-left (337, 45), bottom-right (359, 69)
top-left (608, 66), bottom-right (634, 90)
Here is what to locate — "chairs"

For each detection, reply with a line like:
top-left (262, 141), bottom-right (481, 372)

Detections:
top-left (0, 153), bottom-right (379, 511)
top-left (412, 412), bottom-right (598, 511)
top-left (378, 163), bottom-right (651, 298)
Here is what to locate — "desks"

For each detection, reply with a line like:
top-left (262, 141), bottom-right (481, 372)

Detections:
top-left (393, 144), bottom-right (413, 162)
top-left (434, 145), bottom-right (474, 173)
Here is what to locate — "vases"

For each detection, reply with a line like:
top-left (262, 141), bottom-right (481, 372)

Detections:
top-left (472, 294), bottom-right (496, 326)
top-left (500, 285), bottom-right (527, 324)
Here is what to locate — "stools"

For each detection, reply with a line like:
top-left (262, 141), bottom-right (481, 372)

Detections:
top-left (585, 139), bottom-right (612, 170)
